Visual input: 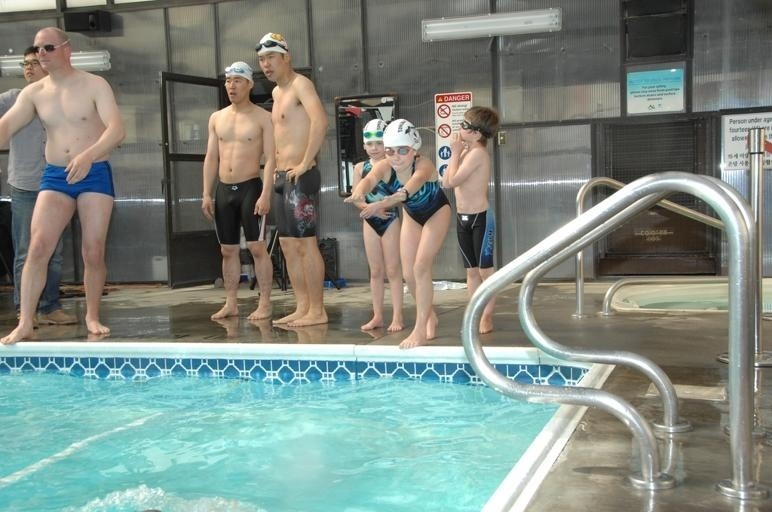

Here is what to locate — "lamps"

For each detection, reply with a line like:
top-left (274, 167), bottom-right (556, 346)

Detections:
top-left (421, 7), bottom-right (562, 42)
top-left (1, 49), bottom-right (113, 79)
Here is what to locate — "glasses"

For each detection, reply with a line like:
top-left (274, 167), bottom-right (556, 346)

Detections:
top-left (223, 65), bottom-right (244, 75)
top-left (363, 131), bottom-right (383, 139)
top-left (460, 120), bottom-right (480, 131)
top-left (19, 60), bottom-right (39, 68)
top-left (32, 44), bottom-right (65, 54)
top-left (383, 147), bottom-right (409, 156)
top-left (256, 40), bottom-right (289, 51)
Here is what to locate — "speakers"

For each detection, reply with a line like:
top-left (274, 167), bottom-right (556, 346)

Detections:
top-left (64, 10), bottom-right (112, 33)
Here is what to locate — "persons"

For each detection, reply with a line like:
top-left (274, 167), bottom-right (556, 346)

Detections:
top-left (343, 119), bottom-right (452, 349)
top-left (0, 46), bottom-right (78, 329)
top-left (255, 33), bottom-right (328, 327)
top-left (200, 61), bottom-right (275, 319)
top-left (351, 119), bottom-right (405, 332)
top-left (0, 27), bottom-right (126, 344)
top-left (442, 106), bottom-right (500, 334)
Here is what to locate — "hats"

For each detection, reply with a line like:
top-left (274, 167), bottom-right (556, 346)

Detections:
top-left (382, 118), bottom-right (422, 151)
top-left (361, 118), bottom-right (388, 145)
top-left (256, 31), bottom-right (289, 56)
top-left (223, 60), bottom-right (255, 82)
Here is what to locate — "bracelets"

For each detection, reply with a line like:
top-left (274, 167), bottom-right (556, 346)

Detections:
top-left (402, 189), bottom-right (409, 203)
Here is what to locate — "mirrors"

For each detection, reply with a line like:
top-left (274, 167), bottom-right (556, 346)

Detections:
top-left (334, 93), bottom-right (399, 197)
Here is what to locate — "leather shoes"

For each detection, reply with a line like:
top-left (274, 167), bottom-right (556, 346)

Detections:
top-left (38, 310), bottom-right (79, 324)
top-left (15, 311), bottom-right (39, 329)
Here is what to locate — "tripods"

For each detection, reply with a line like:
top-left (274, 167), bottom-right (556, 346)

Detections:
top-left (250, 226), bottom-right (340, 292)
